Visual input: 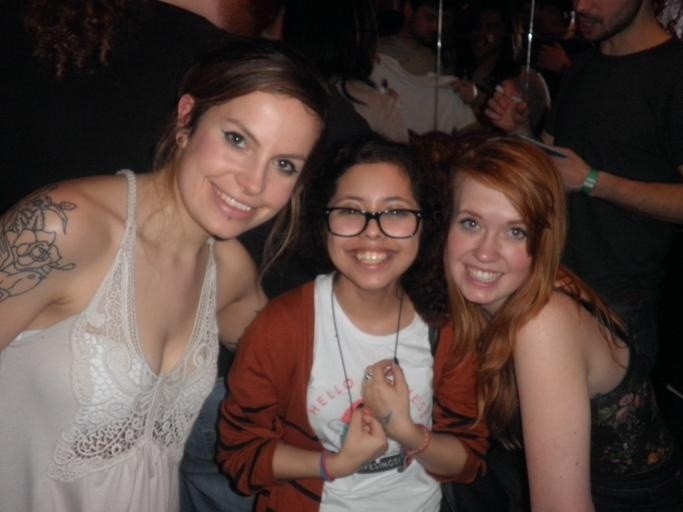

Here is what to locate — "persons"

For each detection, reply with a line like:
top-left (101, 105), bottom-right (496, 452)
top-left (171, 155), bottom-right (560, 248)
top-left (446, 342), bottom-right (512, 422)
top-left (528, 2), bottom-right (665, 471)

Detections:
top-left (0, 1), bottom-right (682, 511)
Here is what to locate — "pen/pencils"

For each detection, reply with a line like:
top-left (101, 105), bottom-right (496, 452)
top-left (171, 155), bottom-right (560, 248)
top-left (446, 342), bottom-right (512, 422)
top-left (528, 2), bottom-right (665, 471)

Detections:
top-left (487, 78), bottom-right (522, 104)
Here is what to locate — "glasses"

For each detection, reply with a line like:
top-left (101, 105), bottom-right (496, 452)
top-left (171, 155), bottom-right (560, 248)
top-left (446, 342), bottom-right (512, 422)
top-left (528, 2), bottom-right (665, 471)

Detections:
top-left (324, 208), bottom-right (423, 238)
